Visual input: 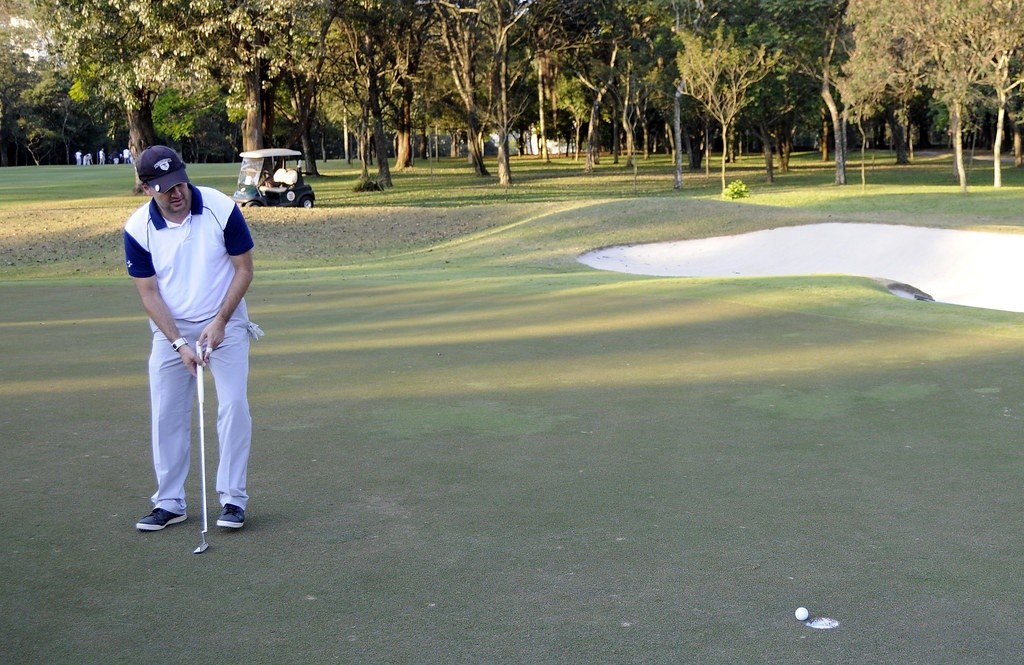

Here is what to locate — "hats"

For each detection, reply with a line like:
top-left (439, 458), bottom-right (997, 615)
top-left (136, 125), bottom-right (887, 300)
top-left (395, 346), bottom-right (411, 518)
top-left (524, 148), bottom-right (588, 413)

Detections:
top-left (136, 145), bottom-right (190, 194)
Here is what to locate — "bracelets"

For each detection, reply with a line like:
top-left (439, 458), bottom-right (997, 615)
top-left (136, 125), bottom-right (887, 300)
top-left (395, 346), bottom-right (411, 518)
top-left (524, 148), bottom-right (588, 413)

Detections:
top-left (170, 336), bottom-right (188, 352)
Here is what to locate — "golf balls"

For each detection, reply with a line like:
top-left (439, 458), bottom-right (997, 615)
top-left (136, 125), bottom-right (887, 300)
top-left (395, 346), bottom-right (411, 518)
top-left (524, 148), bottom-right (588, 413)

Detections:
top-left (795, 606), bottom-right (809, 621)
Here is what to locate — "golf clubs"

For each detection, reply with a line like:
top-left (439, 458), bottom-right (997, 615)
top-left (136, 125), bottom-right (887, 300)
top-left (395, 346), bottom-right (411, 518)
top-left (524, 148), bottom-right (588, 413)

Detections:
top-left (193, 340), bottom-right (209, 554)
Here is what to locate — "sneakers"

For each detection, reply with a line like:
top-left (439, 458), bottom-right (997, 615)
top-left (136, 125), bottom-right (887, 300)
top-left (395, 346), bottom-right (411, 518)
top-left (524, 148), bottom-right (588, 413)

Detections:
top-left (136, 508), bottom-right (187, 531)
top-left (217, 504), bottom-right (245, 527)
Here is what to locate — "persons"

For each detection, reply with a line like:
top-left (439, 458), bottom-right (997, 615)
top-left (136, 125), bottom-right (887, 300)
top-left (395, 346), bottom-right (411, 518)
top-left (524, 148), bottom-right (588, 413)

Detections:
top-left (124, 145), bottom-right (265, 529)
top-left (99, 148), bottom-right (105, 165)
top-left (75, 150), bottom-right (93, 165)
top-left (113, 147), bottom-right (129, 164)
